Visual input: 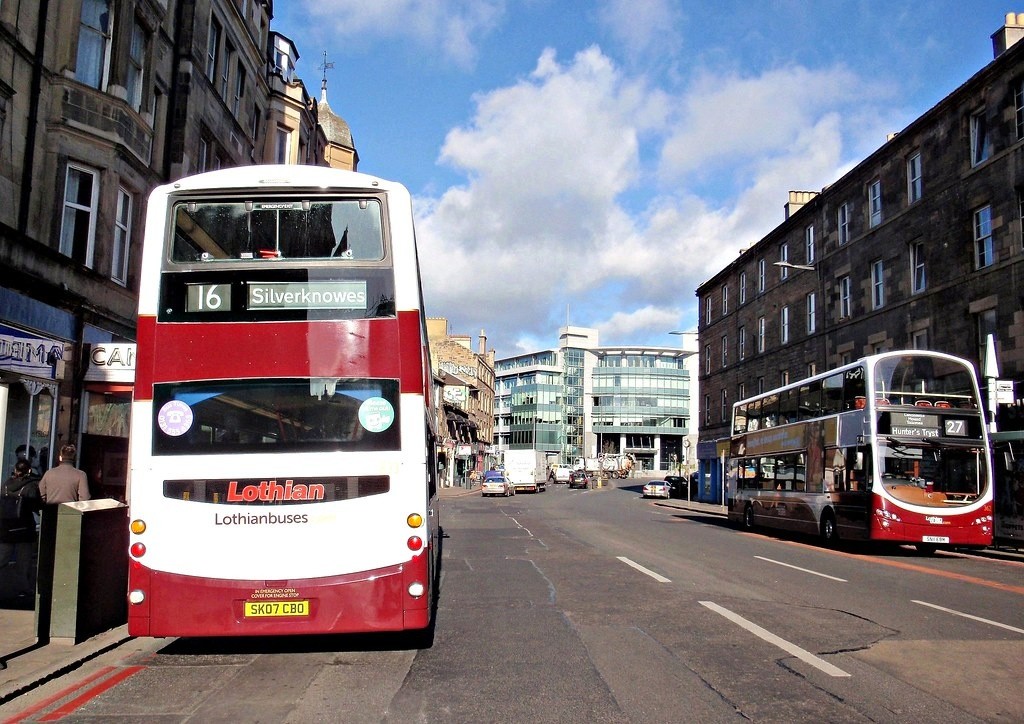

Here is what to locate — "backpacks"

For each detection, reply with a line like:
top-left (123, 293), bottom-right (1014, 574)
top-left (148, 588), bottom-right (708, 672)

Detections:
top-left (0, 478), bottom-right (38, 534)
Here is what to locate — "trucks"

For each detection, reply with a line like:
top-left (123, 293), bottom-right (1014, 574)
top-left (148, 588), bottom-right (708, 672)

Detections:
top-left (504, 449), bottom-right (547, 492)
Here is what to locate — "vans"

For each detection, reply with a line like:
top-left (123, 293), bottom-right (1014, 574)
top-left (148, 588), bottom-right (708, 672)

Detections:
top-left (551, 464), bottom-right (574, 472)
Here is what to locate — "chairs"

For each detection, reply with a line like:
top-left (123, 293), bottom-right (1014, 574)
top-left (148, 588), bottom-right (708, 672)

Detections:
top-left (743, 396), bottom-right (974, 491)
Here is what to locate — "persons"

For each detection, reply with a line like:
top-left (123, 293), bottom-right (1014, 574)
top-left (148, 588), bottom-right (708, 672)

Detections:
top-left (0, 459), bottom-right (44, 597)
top-left (38, 442), bottom-right (92, 504)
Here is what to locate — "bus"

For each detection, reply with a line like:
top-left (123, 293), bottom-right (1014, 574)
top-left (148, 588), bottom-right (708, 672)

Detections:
top-left (725, 349), bottom-right (995, 553)
top-left (127, 164), bottom-right (440, 638)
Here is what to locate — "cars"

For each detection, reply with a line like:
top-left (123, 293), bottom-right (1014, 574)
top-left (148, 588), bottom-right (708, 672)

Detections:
top-left (481, 476), bottom-right (516, 497)
top-left (554, 469), bottom-right (570, 484)
top-left (470, 471), bottom-right (483, 482)
top-left (569, 472), bottom-right (589, 489)
top-left (643, 480), bottom-right (671, 499)
top-left (663, 475), bottom-right (688, 493)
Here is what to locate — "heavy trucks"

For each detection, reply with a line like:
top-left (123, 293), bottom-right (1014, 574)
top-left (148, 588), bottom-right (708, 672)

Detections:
top-left (574, 454), bottom-right (638, 479)
